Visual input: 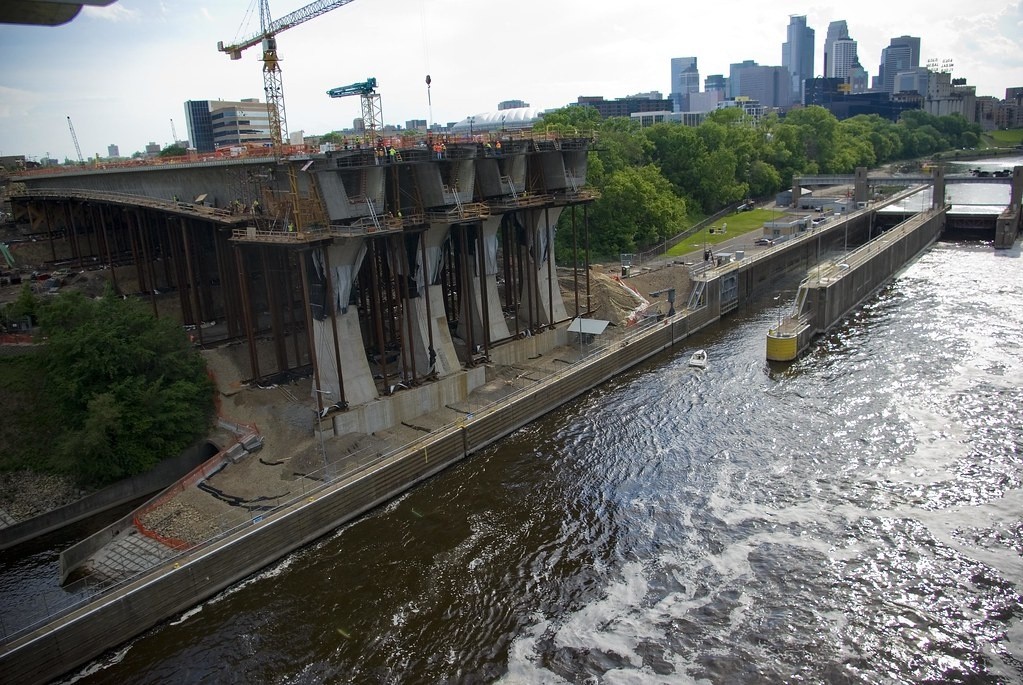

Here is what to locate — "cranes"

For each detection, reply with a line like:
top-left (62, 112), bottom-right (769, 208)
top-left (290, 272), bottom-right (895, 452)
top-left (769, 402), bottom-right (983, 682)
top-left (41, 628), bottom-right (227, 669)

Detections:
top-left (169, 119), bottom-right (178, 144)
top-left (216, 0), bottom-right (356, 157)
top-left (67, 115), bottom-right (85, 168)
top-left (324, 77), bottom-right (385, 147)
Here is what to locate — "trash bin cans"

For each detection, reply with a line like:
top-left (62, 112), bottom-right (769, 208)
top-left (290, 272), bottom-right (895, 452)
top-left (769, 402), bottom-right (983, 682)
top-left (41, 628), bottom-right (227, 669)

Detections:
top-left (622, 266), bottom-right (631, 277)
top-left (705, 251), bottom-right (709, 261)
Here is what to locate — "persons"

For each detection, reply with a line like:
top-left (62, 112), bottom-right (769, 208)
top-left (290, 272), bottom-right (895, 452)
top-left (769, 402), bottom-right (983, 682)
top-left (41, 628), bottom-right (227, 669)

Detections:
top-left (173, 194), bottom-right (177, 204)
top-left (343, 134), bottom-right (396, 165)
top-left (496, 139), bottom-right (501, 156)
top-left (229, 201), bottom-right (233, 211)
top-left (486, 140), bottom-right (492, 155)
top-left (254, 199), bottom-right (259, 212)
top-left (389, 211), bottom-right (394, 219)
top-left (522, 190), bottom-right (526, 196)
top-left (287, 221), bottom-right (293, 233)
top-left (429, 130), bottom-right (446, 159)
top-left (236, 199), bottom-right (240, 212)
top-left (396, 210), bottom-right (403, 218)
top-left (521, 130), bottom-right (525, 138)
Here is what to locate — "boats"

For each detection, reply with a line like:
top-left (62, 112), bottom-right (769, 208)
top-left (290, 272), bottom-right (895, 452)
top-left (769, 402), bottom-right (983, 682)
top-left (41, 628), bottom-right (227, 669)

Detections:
top-left (689, 350), bottom-right (708, 369)
